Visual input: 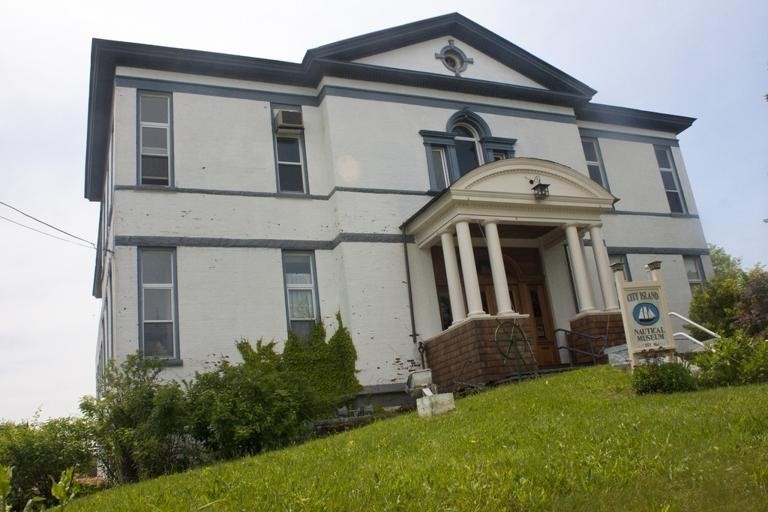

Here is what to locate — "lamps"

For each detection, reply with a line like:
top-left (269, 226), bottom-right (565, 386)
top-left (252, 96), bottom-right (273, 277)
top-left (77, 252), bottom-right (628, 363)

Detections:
top-left (529, 174), bottom-right (550, 200)
top-left (646, 259), bottom-right (662, 272)
